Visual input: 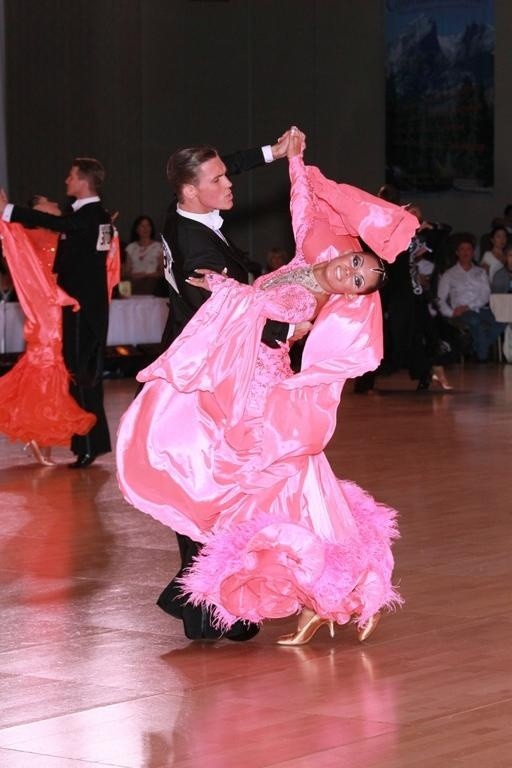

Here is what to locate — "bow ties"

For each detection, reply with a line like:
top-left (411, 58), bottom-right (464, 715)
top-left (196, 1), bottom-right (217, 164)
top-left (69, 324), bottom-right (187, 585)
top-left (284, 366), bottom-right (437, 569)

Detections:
top-left (213, 217), bottom-right (224, 230)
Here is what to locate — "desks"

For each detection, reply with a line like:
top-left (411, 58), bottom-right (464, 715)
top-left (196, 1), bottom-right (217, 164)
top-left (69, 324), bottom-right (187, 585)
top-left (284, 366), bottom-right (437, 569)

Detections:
top-left (0, 296), bottom-right (171, 355)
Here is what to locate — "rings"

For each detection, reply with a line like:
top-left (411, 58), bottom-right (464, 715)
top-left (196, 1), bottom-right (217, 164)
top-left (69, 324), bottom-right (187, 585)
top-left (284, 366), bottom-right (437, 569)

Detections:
top-left (198, 277), bottom-right (204, 282)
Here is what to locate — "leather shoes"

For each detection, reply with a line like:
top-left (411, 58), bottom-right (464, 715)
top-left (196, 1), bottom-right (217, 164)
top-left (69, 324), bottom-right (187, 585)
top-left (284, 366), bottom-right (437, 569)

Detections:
top-left (416, 379), bottom-right (430, 392)
top-left (68, 451), bottom-right (97, 468)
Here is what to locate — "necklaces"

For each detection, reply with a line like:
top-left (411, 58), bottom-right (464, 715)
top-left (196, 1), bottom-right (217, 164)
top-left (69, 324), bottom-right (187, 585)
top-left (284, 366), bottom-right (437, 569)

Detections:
top-left (261, 264), bottom-right (330, 296)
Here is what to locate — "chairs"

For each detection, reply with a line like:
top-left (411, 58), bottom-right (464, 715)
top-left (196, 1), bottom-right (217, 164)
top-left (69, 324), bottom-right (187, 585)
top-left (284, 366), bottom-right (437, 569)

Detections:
top-left (488, 287), bottom-right (512, 365)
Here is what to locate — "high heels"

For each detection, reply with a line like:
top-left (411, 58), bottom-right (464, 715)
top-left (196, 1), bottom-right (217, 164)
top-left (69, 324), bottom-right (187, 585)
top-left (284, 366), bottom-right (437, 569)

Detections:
top-left (275, 613), bottom-right (335, 646)
top-left (356, 608), bottom-right (383, 641)
top-left (432, 374), bottom-right (454, 391)
top-left (23, 439), bottom-right (57, 466)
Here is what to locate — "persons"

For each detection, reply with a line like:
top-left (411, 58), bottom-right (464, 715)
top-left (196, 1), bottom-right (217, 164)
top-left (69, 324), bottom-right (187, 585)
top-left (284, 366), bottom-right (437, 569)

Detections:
top-left (355, 207), bottom-right (512, 393)
top-left (117, 215), bottom-right (287, 379)
top-left (157, 135), bottom-right (314, 642)
top-left (185, 125), bottom-right (388, 646)
top-left (4, 156), bottom-right (123, 469)
